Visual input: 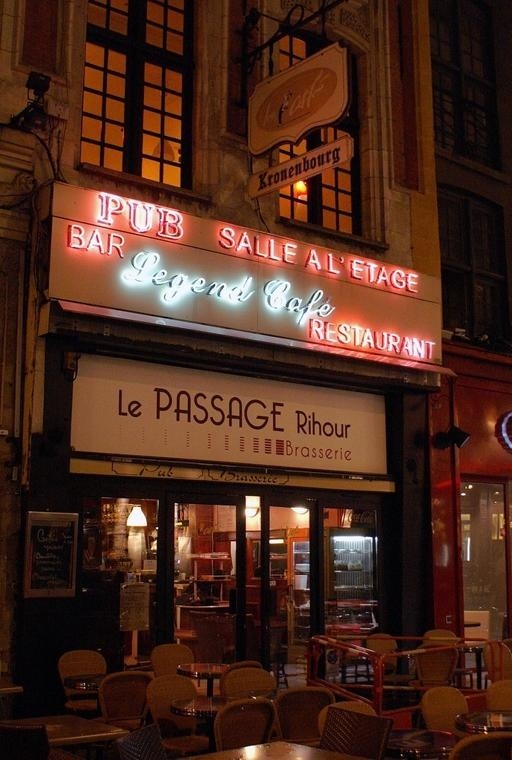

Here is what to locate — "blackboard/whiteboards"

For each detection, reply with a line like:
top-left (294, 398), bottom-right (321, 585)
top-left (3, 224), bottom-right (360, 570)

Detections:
top-left (24, 511), bottom-right (79, 598)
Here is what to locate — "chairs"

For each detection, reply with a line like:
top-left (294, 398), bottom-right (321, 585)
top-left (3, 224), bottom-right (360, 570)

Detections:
top-left (1, 607), bottom-right (512, 760)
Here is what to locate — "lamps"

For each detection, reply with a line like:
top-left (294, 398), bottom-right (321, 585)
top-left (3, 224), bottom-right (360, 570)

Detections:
top-left (124, 504), bottom-right (149, 528)
top-left (6, 69), bottom-right (53, 127)
top-left (289, 506), bottom-right (309, 515)
top-left (245, 507), bottom-right (261, 518)
top-left (433, 424), bottom-right (470, 450)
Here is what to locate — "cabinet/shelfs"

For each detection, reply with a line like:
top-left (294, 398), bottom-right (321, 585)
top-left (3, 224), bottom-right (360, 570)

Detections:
top-left (287, 526), bottom-right (376, 669)
